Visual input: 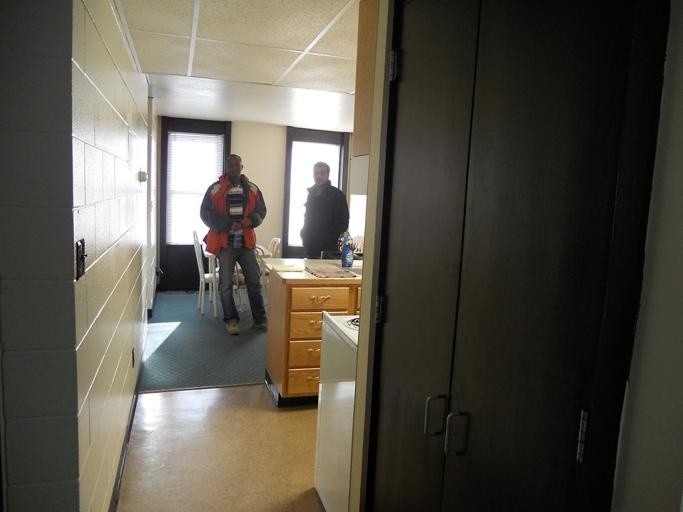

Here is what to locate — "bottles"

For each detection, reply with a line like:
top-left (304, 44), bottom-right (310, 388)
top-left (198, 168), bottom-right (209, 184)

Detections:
top-left (232, 224), bottom-right (242, 249)
top-left (340, 231), bottom-right (353, 270)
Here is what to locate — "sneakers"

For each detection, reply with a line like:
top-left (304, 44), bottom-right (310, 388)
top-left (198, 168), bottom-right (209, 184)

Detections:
top-left (254, 321), bottom-right (267, 331)
top-left (225, 318), bottom-right (240, 335)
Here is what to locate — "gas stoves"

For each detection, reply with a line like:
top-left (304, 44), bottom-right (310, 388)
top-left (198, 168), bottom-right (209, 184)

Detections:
top-left (330, 316), bottom-right (359, 347)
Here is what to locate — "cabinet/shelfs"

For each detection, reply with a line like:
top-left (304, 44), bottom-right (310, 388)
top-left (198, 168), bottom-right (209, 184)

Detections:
top-left (349, 0), bottom-right (380, 160)
top-left (260, 267), bottom-right (362, 410)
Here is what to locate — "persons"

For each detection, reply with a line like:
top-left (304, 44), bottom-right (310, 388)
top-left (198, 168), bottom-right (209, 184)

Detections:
top-left (199, 152), bottom-right (268, 336)
top-left (298, 162), bottom-right (349, 259)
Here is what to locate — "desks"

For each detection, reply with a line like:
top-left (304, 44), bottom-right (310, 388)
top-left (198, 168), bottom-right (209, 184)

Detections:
top-left (314, 301), bottom-right (367, 511)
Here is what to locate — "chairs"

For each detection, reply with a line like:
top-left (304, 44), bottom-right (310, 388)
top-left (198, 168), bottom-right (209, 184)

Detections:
top-left (187, 232), bottom-right (283, 321)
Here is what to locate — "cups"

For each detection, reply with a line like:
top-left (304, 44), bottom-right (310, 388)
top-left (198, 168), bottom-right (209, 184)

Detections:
top-left (319, 247), bottom-right (329, 260)
